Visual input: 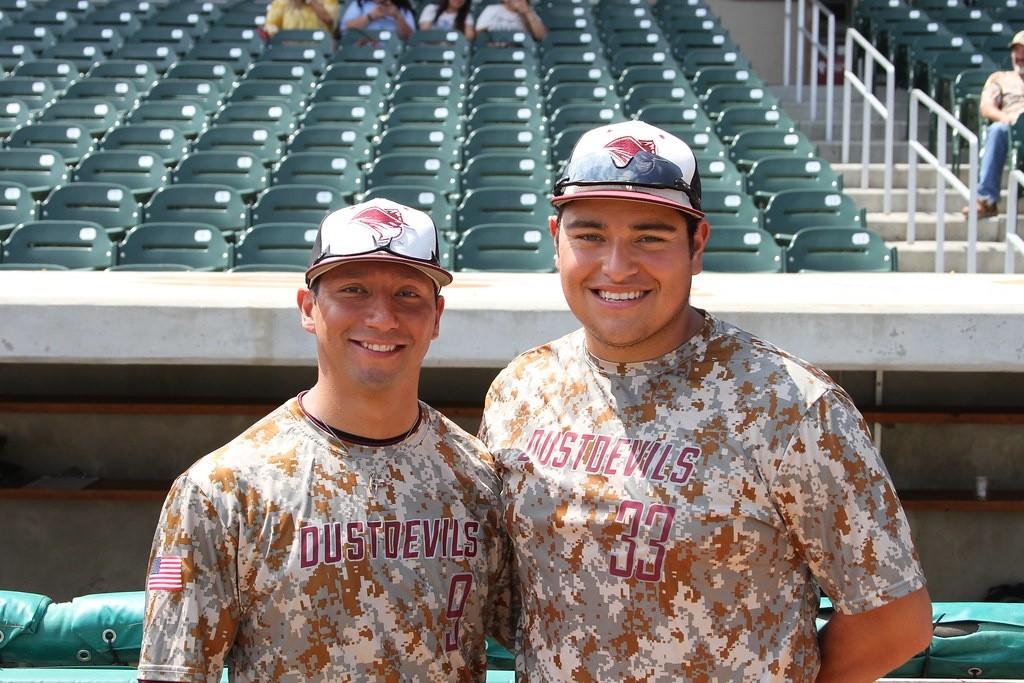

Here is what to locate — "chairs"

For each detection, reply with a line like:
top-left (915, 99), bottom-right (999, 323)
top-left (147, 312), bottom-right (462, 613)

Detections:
top-left (0, 0), bottom-right (1023, 275)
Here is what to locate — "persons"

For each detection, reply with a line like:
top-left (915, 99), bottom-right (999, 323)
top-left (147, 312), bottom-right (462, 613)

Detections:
top-left (265, 0), bottom-right (339, 46)
top-left (418, 0), bottom-right (475, 46)
top-left (477, 119), bottom-right (934, 683)
top-left (340, 0), bottom-right (416, 46)
top-left (963, 29), bottom-right (1024, 218)
top-left (137, 198), bottom-right (516, 683)
top-left (475, 0), bottom-right (546, 47)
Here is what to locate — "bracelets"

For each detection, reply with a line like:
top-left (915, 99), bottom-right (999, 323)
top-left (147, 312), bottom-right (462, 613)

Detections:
top-left (367, 13), bottom-right (373, 21)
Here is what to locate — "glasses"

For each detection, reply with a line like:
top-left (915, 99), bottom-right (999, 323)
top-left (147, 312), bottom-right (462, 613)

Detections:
top-left (310, 224), bottom-right (439, 264)
top-left (553, 149), bottom-right (702, 212)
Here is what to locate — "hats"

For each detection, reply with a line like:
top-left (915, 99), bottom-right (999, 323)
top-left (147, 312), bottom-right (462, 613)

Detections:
top-left (305, 197), bottom-right (452, 290)
top-left (1008, 30), bottom-right (1024, 47)
top-left (551, 121), bottom-right (707, 220)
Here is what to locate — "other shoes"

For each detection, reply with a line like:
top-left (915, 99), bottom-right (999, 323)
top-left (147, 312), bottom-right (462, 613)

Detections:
top-left (962, 200), bottom-right (997, 220)
